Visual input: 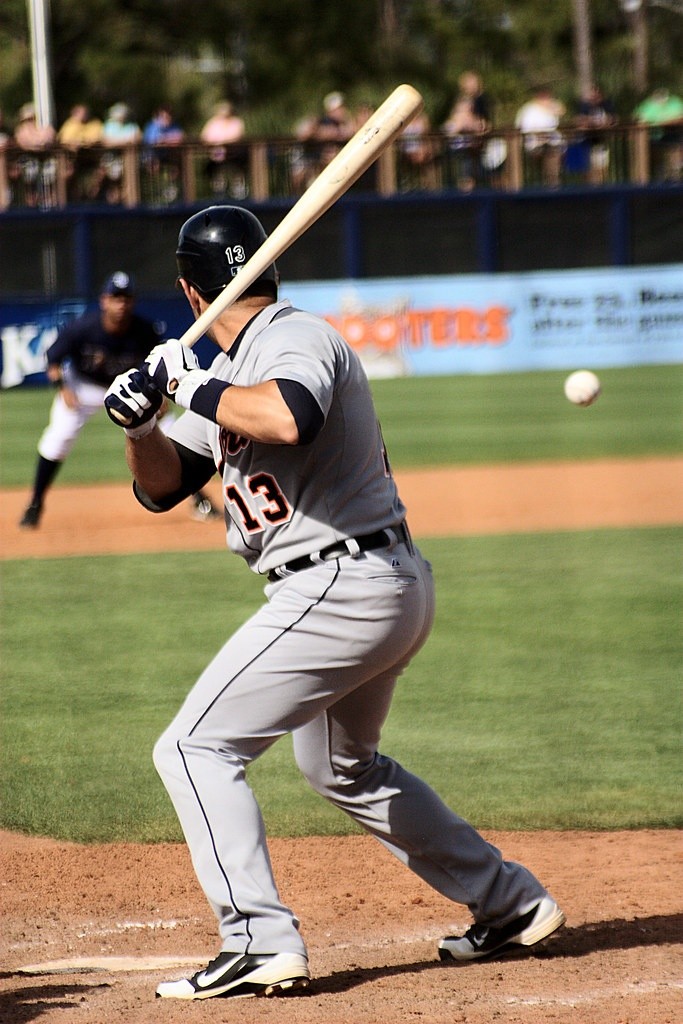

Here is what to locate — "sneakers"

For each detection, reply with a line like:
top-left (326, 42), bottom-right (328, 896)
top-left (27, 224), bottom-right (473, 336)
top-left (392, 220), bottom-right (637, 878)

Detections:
top-left (438, 893), bottom-right (568, 963)
top-left (192, 501), bottom-right (223, 523)
top-left (20, 504), bottom-right (43, 530)
top-left (156, 951), bottom-right (311, 999)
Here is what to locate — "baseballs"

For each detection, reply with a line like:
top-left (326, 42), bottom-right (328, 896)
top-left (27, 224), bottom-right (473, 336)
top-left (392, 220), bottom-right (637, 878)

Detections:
top-left (563, 369), bottom-right (600, 408)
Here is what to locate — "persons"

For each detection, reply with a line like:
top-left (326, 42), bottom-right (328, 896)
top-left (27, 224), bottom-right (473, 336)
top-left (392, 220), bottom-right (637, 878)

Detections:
top-left (0, 71), bottom-right (682, 210)
top-left (18, 267), bottom-right (224, 524)
top-left (99, 209), bottom-right (570, 1001)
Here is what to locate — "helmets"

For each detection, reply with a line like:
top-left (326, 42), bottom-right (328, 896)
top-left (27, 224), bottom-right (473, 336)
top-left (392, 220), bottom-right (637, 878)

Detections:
top-left (101, 271), bottom-right (135, 298)
top-left (175, 207), bottom-right (281, 298)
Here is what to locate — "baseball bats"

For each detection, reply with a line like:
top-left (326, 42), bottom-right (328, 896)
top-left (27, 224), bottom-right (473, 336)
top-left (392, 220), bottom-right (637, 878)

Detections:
top-left (177, 83), bottom-right (423, 351)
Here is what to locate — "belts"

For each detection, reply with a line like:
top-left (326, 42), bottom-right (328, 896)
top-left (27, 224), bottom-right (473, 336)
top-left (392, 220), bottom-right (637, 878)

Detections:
top-left (267, 522), bottom-right (408, 581)
